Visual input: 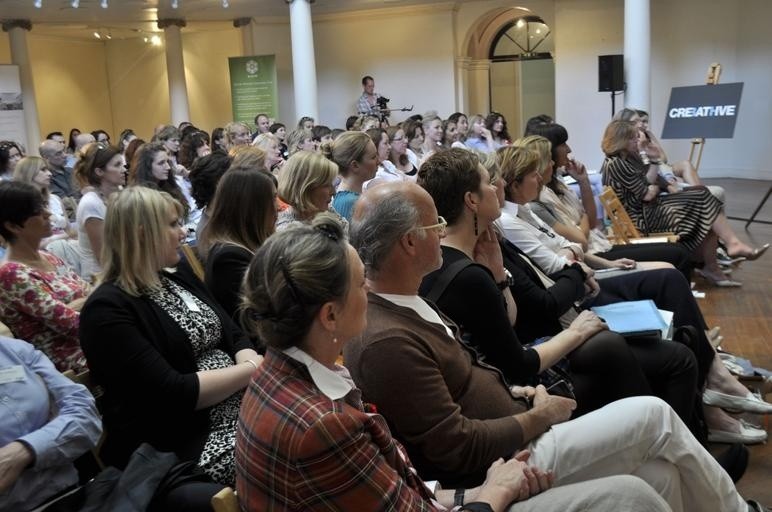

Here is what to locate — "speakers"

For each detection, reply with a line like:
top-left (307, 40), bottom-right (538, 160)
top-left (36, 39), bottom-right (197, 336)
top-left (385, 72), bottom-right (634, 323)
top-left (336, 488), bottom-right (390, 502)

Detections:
top-left (598, 54), bottom-right (622, 91)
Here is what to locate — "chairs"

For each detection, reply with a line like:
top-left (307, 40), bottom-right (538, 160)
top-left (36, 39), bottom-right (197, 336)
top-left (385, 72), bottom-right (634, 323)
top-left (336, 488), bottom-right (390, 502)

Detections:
top-left (598, 185), bottom-right (680, 244)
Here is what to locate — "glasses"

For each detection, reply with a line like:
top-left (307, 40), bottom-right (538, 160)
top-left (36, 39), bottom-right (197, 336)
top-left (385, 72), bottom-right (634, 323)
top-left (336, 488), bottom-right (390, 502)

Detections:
top-left (416, 215), bottom-right (448, 234)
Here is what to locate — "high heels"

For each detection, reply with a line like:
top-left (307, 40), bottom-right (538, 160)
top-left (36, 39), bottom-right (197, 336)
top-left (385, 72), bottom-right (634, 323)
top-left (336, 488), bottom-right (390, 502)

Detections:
top-left (728, 242), bottom-right (770, 264)
top-left (700, 268), bottom-right (744, 288)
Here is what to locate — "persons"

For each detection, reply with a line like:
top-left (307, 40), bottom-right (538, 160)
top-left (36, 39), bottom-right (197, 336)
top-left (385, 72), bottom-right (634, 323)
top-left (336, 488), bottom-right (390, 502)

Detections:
top-left (1, 109), bottom-right (771, 512)
top-left (355, 75), bottom-right (391, 123)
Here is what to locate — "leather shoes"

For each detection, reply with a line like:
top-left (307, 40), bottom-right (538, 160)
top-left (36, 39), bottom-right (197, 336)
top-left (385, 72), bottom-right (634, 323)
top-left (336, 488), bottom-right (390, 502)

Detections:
top-left (701, 387), bottom-right (772, 416)
top-left (706, 416), bottom-right (768, 446)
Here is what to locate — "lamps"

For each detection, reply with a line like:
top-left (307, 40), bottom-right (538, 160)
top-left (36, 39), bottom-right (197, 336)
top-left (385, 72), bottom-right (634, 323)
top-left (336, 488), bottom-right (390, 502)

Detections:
top-left (172, 0), bottom-right (178, 8)
top-left (223, 0), bottom-right (229, 8)
top-left (100, 0), bottom-right (108, 9)
top-left (516, 19), bottom-right (524, 28)
top-left (72, 0), bottom-right (80, 9)
top-left (34, 0), bottom-right (42, 9)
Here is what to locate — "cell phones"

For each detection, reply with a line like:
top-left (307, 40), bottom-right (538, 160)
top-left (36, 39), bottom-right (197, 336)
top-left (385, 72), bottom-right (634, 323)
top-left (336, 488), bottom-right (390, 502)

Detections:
top-left (530, 381), bottom-right (575, 408)
